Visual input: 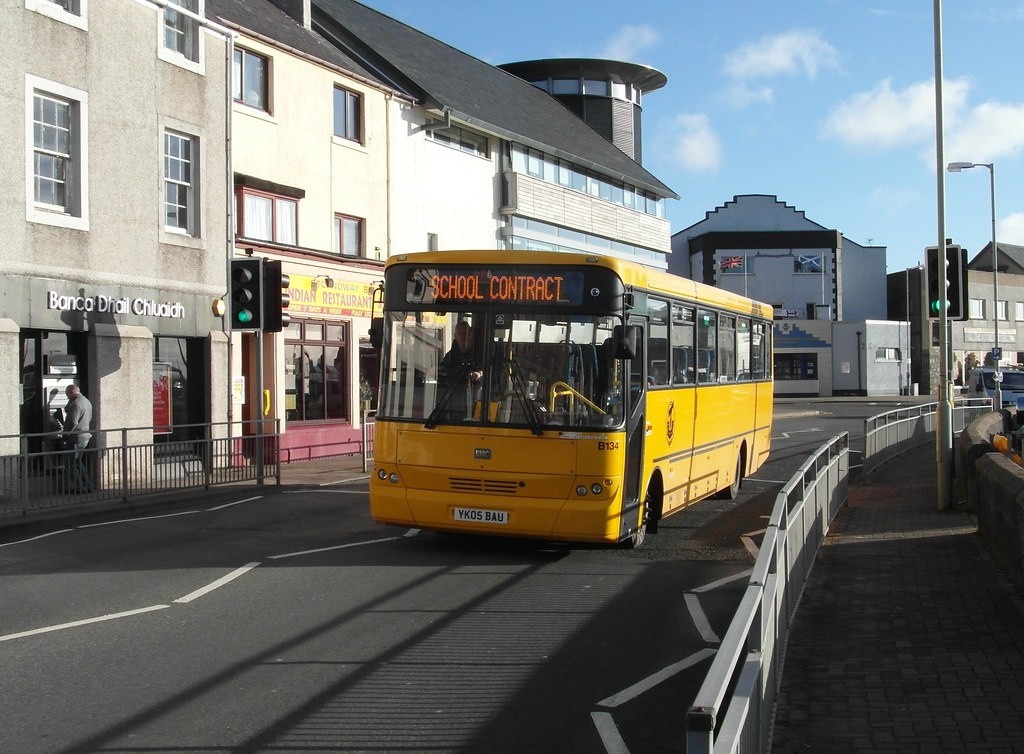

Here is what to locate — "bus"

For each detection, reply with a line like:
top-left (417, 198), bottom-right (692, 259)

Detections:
top-left (365, 248), bottom-right (775, 550)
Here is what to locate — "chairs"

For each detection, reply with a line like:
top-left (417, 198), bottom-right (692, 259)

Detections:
top-left (719, 348), bottom-right (729, 381)
top-left (628, 337), bottom-right (670, 388)
top-left (686, 348), bottom-right (710, 383)
top-left (727, 350), bottom-right (738, 381)
top-left (673, 349), bottom-right (688, 383)
top-left (707, 349), bottom-right (722, 382)
top-left (540, 340), bottom-right (600, 400)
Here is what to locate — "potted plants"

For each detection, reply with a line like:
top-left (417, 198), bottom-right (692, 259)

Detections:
top-left (360, 375), bottom-right (372, 413)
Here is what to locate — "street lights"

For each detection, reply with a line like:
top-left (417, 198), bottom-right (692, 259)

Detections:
top-left (947, 161), bottom-right (1000, 410)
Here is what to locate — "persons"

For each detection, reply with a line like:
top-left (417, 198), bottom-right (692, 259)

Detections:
top-left (428, 320), bottom-right (489, 419)
top-left (64, 385), bottom-right (93, 493)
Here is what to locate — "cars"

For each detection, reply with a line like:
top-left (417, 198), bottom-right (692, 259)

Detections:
top-left (967, 366), bottom-right (1024, 411)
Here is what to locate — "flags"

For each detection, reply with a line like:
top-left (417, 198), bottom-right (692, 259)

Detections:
top-left (797, 255), bottom-right (822, 273)
top-left (720, 255), bottom-right (744, 269)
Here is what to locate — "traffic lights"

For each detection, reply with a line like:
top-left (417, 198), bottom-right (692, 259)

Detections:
top-left (701, 248), bottom-right (716, 286)
top-left (262, 260), bottom-right (289, 333)
top-left (229, 258), bottom-right (261, 326)
top-left (927, 248), bottom-right (960, 317)
